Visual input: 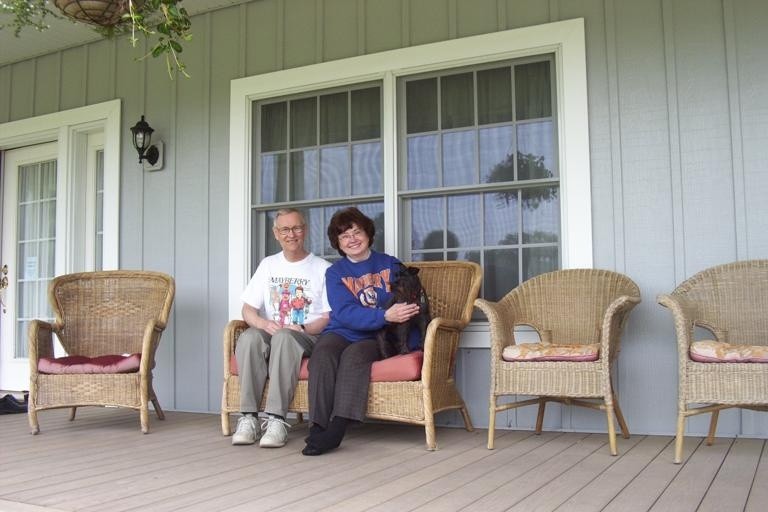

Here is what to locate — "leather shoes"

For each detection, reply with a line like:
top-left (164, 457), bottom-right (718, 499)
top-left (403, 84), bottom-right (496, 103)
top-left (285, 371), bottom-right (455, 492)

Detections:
top-left (0, 394), bottom-right (28, 415)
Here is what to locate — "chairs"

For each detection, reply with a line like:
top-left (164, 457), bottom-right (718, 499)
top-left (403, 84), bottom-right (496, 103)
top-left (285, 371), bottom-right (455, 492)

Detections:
top-left (27, 270), bottom-right (176, 435)
top-left (657, 260), bottom-right (768, 464)
top-left (474, 268), bottom-right (642, 456)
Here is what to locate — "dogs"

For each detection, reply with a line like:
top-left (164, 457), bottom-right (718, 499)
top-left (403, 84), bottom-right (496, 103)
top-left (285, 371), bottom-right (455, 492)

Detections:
top-left (376, 266), bottom-right (432, 359)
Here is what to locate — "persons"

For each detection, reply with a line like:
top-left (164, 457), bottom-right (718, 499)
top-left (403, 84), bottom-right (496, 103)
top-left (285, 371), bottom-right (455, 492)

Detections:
top-left (232, 208), bottom-right (333, 448)
top-left (300, 207), bottom-right (420, 456)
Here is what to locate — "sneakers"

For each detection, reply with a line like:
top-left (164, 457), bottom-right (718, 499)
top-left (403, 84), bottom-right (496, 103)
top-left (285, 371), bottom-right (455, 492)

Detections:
top-left (260, 417), bottom-right (291, 447)
top-left (232, 416), bottom-right (262, 444)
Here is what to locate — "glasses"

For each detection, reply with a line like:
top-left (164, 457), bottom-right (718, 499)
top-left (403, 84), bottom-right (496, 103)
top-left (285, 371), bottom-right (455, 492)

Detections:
top-left (336, 227), bottom-right (365, 241)
top-left (277, 225), bottom-right (304, 234)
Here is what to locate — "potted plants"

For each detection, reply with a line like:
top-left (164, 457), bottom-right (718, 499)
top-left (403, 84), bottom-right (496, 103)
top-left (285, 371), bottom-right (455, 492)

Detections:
top-left (0, 0), bottom-right (193, 80)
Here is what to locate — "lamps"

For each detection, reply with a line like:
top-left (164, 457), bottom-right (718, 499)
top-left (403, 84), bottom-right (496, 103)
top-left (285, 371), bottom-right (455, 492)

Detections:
top-left (130, 116), bottom-right (164, 172)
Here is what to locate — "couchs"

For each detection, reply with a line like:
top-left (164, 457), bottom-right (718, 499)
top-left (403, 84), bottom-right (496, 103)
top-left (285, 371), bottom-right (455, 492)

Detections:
top-left (221, 261), bottom-right (482, 450)
